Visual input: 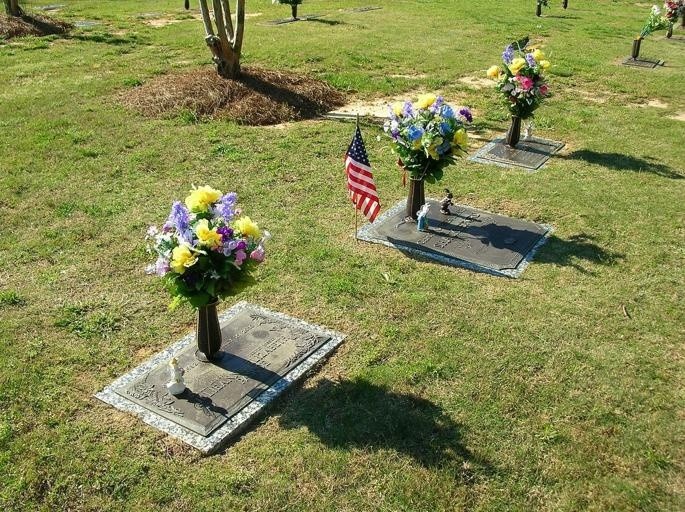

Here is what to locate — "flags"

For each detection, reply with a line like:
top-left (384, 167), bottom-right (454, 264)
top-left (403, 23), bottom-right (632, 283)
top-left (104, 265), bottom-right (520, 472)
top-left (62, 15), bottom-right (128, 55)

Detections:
top-left (342, 126), bottom-right (381, 225)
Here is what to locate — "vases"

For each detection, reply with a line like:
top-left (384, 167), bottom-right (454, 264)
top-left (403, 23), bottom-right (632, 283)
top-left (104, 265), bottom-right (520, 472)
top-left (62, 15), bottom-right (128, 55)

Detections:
top-left (506, 112), bottom-right (520, 148)
top-left (632, 40), bottom-right (641, 58)
top-left (405, 176), bottom-right (425, 221)
top-left (535, 3), bottom-right (541, 17)
top-left (195, 306), bottom-right (222, 362)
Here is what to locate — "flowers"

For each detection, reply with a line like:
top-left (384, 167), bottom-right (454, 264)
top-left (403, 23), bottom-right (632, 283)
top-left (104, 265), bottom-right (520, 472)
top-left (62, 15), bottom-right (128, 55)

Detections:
top-left (487, 37), bottom-right (553, 121)
top-left (637, 1), bottom-right (682, 41)
top-left (376, 95), bottom-right (473, 185)
top-left (144, 183), bottom-right (272, 308)
